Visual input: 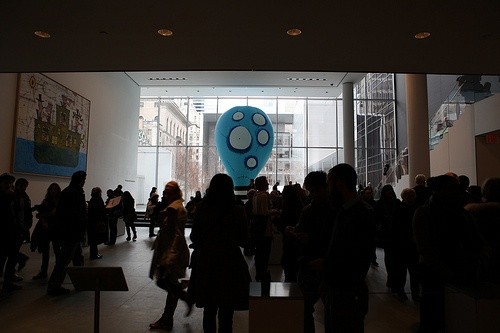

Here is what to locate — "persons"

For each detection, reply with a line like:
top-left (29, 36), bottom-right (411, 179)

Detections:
top-left (0, 162), bottom-right (500, 333)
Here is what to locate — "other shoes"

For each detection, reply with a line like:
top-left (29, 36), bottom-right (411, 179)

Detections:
top-left (149, 314), bottom-right (174, 331)
top-left (90, 254), bottom-right (104, 259)
top-left (17, 254), bottom-right (30, 269)
top-left (48, 285), bottom-right (72, 296)
top-left (32, 272), bottom-right (49, 279)
top-left (126, 235), bottom-right (138, 240)
top-left (0, 273), bottom-right (23, 289)
top-left (185, 302), bottom-right (194, 317)
top-left (149, 233), bottom-right (157, 237)
